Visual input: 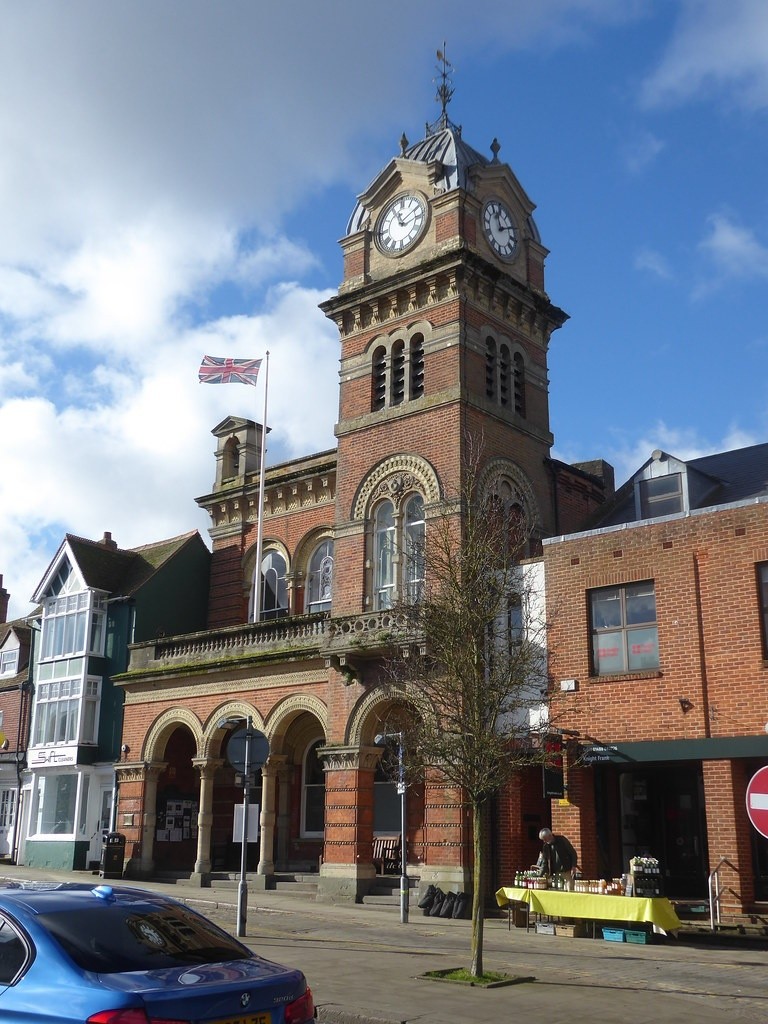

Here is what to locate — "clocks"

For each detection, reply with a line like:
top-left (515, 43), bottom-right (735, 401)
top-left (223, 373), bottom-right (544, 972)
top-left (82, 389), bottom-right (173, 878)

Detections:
top-left (480, 193), bottom-right (521, 264)
top-left (372, 189), bottom-right (430, 259)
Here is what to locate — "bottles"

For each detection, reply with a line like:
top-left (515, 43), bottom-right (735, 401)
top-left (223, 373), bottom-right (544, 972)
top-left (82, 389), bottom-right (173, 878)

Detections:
top-left (514, 854), bottom-right (661, 898)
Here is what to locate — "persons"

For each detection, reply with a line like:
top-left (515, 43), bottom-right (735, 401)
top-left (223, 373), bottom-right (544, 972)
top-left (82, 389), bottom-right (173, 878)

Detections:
top-left (536, 828), bottom-right (578, 881)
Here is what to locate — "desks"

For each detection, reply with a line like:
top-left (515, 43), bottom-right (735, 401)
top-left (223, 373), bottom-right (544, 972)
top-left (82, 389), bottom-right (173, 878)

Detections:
top-left (495, 886), bottom-right (683, 944)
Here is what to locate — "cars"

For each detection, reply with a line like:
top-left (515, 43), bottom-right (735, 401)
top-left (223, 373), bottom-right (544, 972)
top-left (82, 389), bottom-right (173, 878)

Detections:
top-left (0, 880), bottom-right (317, 1024)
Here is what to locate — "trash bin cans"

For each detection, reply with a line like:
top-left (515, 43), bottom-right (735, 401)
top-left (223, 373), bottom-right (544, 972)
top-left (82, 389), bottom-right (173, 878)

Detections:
top-left (99, 833), bottom-right (126, 880)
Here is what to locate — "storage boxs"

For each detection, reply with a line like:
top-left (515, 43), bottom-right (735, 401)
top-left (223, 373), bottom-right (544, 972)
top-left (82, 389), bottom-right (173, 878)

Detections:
top-left (554, 923), bottom-right (584, 937)
top-left (602, 925), bottom-right (626, 942)
top-left (535, 922), bottom-right (555, 935)
top-left (624, 929), bottom-right (658, 945)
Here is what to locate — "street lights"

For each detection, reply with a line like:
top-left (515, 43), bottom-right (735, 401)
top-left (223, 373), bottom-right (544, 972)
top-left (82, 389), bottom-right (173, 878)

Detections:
top-left (218, 713), bottom-right (253, 939)
top-left (375, 730), bottom-right (410, 924)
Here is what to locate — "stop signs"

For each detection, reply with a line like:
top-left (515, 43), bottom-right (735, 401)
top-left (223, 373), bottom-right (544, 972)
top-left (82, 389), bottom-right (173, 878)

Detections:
top-left (745, 764), bottom-right (767, 839)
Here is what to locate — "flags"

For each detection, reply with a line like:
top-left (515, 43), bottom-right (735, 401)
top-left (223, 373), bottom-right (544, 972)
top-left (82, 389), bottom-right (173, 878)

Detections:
top-left (198, 356), bottom-right (263, 386)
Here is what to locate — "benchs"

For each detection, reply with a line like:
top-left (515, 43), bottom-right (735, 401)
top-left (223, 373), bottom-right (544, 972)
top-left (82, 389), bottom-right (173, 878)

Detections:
top-left (373, 835), bottom-right (402, 875)
